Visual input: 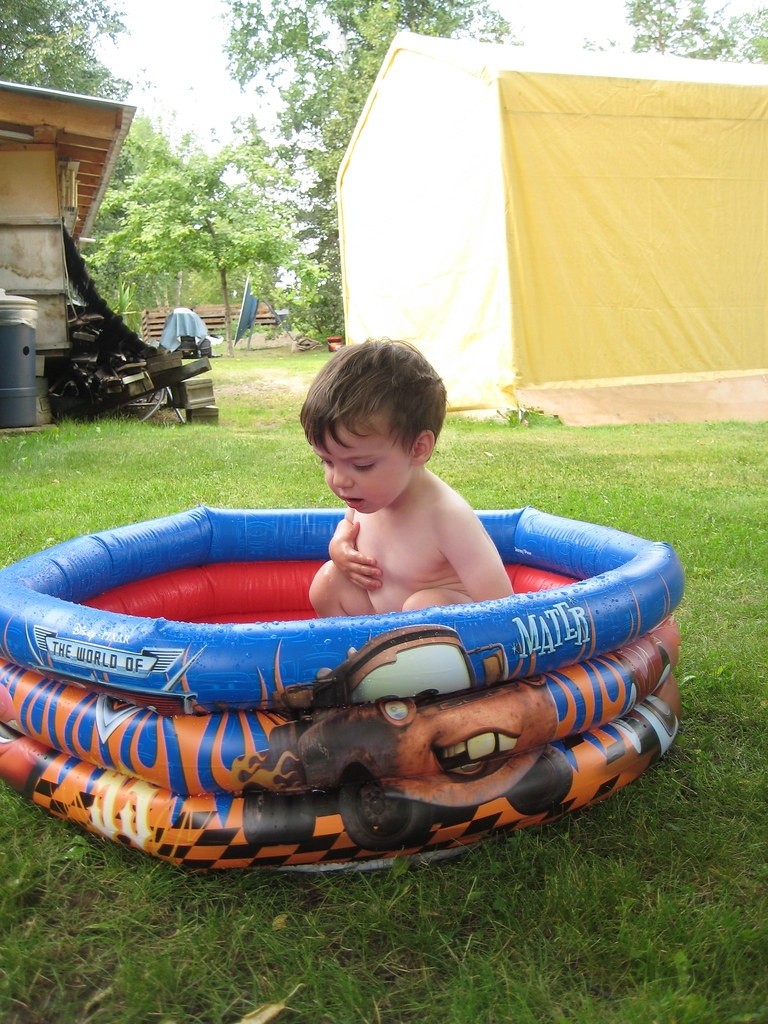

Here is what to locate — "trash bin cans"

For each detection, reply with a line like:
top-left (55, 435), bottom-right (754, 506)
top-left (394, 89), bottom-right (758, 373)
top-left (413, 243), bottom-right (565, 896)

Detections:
top-left (0, 294), bottom-right (41, 428)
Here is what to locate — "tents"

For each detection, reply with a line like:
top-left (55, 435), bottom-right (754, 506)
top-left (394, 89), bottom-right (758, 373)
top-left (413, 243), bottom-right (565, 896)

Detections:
top-left (334, 30), bottom-right (768, 426)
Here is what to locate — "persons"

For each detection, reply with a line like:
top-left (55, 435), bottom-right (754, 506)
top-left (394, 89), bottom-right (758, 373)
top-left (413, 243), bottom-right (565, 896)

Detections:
top-left (299, 335), bottom-right (515, 619)
